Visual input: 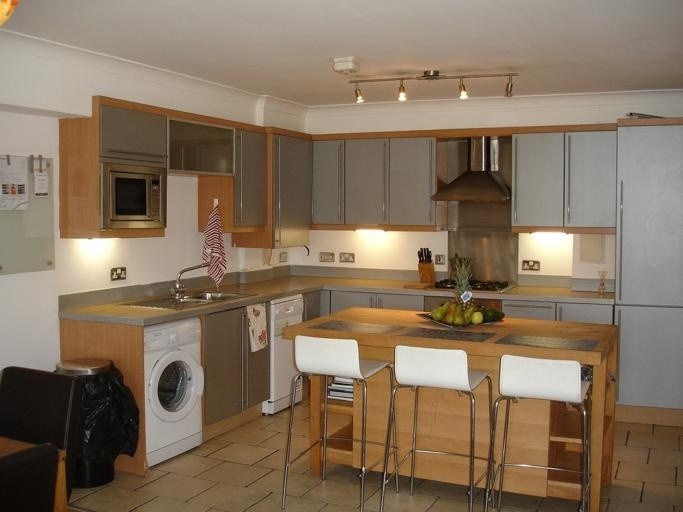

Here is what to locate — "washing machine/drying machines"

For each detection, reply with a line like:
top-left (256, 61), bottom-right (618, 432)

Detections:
top-left (59, 301), bottom-right (204, 477)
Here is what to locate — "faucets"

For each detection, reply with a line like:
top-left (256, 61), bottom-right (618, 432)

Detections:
top-left (175, 260), bottom-right (214, 300)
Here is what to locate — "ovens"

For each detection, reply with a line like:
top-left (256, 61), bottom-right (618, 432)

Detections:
top-left (423, 295), bottom-right (501, 315)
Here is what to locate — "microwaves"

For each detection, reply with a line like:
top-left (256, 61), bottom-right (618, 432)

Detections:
top-left (100, 167), bottom-right (166, 231)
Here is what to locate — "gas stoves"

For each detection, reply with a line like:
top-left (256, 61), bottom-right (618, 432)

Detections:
top-left (424, 278), bottom-right (516, 293)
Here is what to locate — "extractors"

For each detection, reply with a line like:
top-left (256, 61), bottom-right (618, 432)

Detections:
top-left (430, 136), bottom-right (511, 203)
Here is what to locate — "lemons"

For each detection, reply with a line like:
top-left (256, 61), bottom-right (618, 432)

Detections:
top-left (471, 311), bottom-right (483, 324)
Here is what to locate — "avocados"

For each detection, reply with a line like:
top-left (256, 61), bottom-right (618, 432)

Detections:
top-left (484, 309), bottom-right (505, 323)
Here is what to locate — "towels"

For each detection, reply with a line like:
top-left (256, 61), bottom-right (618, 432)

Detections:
top-left (201, 206), bottom-right (226, 289)
top-left (246, 303), bottom-right (267, 352)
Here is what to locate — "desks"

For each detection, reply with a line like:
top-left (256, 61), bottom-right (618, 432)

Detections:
top-left (282, 306), bottom-right (620, 511)
top-left (0, 437), bottom-right (68, 512)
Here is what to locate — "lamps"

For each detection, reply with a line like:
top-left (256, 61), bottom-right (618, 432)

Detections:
top-left (347, 73), bottom-right (518, 103)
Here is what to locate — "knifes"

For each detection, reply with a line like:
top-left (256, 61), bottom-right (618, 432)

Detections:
top-left (416, 246), bottom-right (432, 264)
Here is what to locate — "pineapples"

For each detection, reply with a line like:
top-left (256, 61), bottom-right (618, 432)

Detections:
top-left (454, 259), bottom-right (476, 311)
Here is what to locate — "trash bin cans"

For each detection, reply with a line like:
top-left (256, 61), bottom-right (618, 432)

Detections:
top-left (56, 358), bottom-right (114, 488)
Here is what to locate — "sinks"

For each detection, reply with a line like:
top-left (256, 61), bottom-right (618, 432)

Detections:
top-left (180, 288), bottom-right (243, 301)
top-left (123, 299), bottom-right (206, 311)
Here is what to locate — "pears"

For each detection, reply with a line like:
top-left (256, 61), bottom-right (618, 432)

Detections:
top-left (431, 302), bottom-right (474, 325)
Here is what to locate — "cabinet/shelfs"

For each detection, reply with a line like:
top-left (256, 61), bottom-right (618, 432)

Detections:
top-left (303, 288), bottom-right (321, 322)
top-left (58, 96), bottom-right (169, 240)
top-left (512, 129), bottom-right (616, 236)
top-left (388, 129), bottom-right (445, 234)
top-left (309, 136), bottom-right (388, 232)
top-left (502, 299), bottom-right (554, 323)
top-left (331, 288), bottom-right (424, 314)
top-left (200, 302), bottom-right (270, 443)
top-left (229, 125), bottom-right (310, 248)
top-left (614, 117), bottom-right (682, 427)
top-left (198, 125), bottom-right (265, 234)
top-left (557, 302), bottom-right (613, 324)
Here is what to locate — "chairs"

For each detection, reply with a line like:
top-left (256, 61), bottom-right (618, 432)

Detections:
top-left (380, 343), bottom-right (492, 512)
top-left (0, 365), bottom-right (79, 500)
top-left (0, 443), bottom-right (59, 512)
top-left (479, 355), bottom-right (596, 512)
top-left (276, 334), bottom-right (394, 511)
